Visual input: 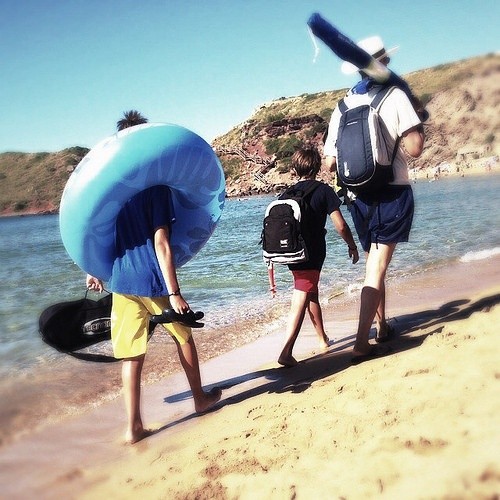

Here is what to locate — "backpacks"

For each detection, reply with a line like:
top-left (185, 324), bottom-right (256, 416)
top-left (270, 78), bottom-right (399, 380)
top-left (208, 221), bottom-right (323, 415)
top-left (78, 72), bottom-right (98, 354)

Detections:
top-left (335, 84), bottom-right (397, 190)
top-left (259, 181), bottom-right (323, 265)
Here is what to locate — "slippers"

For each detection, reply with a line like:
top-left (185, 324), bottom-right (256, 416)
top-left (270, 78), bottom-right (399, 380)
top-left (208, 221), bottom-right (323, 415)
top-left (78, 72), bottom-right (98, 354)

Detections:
top-left (375, 325), bottom-right (395, 342)
top-left (350, 343), bottom-right (376, 364)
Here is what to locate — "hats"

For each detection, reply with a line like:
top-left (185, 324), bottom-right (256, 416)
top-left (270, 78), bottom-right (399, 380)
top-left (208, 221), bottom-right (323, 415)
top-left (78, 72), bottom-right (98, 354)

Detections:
top-left (340, 37), bottom-right (398, 73)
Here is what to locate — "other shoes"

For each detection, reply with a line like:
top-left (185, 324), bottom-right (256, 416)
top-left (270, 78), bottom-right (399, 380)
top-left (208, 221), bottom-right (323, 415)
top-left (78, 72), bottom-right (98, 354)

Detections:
top-left (152, 308), bottom-right (204, 329)
top-left (325, 337), bottom-right (334, 348)
top-left (276, 358), bottom-right (304, 370)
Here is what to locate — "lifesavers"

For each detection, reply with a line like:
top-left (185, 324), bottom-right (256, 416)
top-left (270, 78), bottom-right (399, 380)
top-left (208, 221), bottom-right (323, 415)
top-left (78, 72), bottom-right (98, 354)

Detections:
top-left (58, 122), bottom-right (226, 282)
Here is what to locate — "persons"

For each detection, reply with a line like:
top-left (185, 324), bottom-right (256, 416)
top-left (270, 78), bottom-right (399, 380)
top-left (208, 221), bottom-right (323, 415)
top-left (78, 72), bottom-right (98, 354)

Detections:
top-left (263, 150), bottom-right (361, 366)
top-left (81, 108), bottom-right (223, 445)
top-left (322, 35), bottom-right (426, 357)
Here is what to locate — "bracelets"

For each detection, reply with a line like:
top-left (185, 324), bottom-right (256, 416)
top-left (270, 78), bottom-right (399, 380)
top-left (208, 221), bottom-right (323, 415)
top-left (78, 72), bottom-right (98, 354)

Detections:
top-left (348, 245), bottom-right (357, 250)
top-left (168, 288), bottom-right (181, 297)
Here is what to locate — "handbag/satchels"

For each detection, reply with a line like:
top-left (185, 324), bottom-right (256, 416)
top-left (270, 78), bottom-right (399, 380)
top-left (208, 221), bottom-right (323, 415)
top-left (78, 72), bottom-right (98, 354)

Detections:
top-left (37, 284), bottom-right (157, 364)
